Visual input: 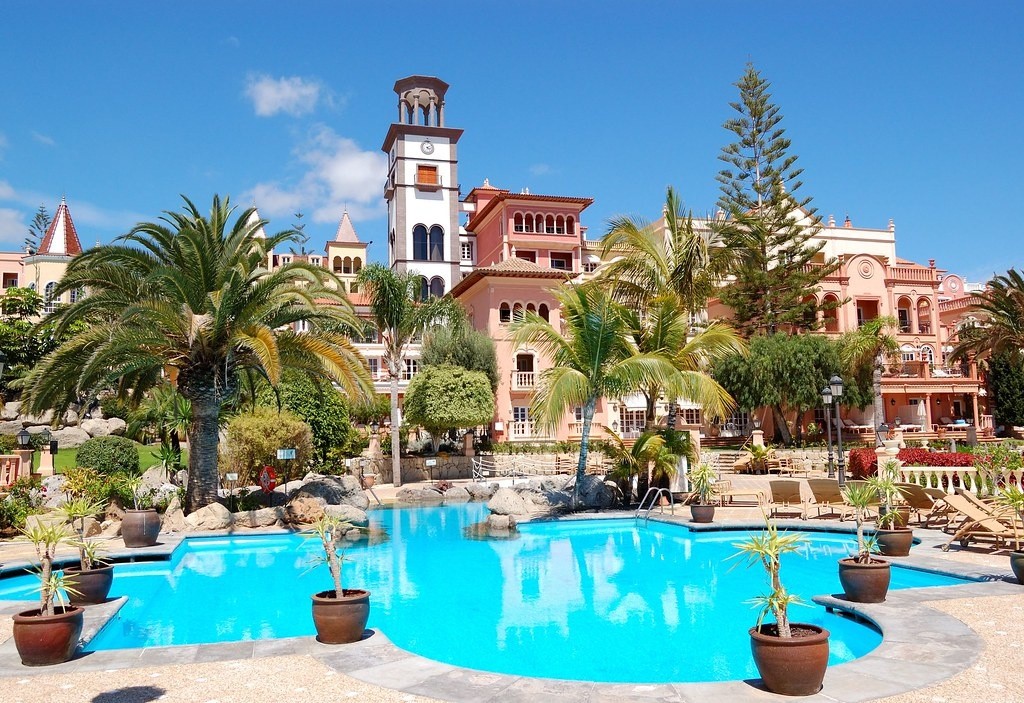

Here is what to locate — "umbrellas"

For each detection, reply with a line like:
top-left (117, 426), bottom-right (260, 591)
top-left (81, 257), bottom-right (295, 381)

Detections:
top-left (917, 400), bottom-right (927, 416)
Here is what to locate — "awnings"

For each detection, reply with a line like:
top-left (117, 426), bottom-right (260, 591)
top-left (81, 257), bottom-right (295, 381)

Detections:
top-left (621, 395), bottom-right (662, 412)
top-left (676, 399), bottom-right (703, 410)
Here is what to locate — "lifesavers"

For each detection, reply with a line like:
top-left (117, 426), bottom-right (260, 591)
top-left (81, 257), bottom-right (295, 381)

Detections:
top-left (260, 466), bottom-right (276, 492)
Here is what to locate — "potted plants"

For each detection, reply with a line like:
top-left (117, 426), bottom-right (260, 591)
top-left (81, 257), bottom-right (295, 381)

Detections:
top-left (295, 508), bottom-right (371, 645)
top-left (860, 459), bottom-right (913, 557)
top-left (51, 498), bottom-right (114, 606)
top-left (723, 494), bottom-right (831, 696)
top-left (116, 472), bottom-right (161, 549)
top-left (997, 482), bottom-right (1024, 584)
top-left (838, 483), bottom-right (891, 603)
top-left (689, 466), bottom-right (718, 523)
top-left (11, 514), bottom-right (86, 668)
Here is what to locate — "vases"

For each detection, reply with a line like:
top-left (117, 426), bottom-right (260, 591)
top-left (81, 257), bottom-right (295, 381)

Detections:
top-left (362, 474), bottom-right (375, 488)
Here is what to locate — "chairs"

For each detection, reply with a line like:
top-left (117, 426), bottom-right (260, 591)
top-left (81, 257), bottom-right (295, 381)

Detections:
top-left (766, 458), bottom-right (810, 479)
top-left (882, 366), bottom-right (962, 378)
top-left (769, 480), bottom-right (1024, 553)
top-left (832, 415), bottom-right (970, 434)
